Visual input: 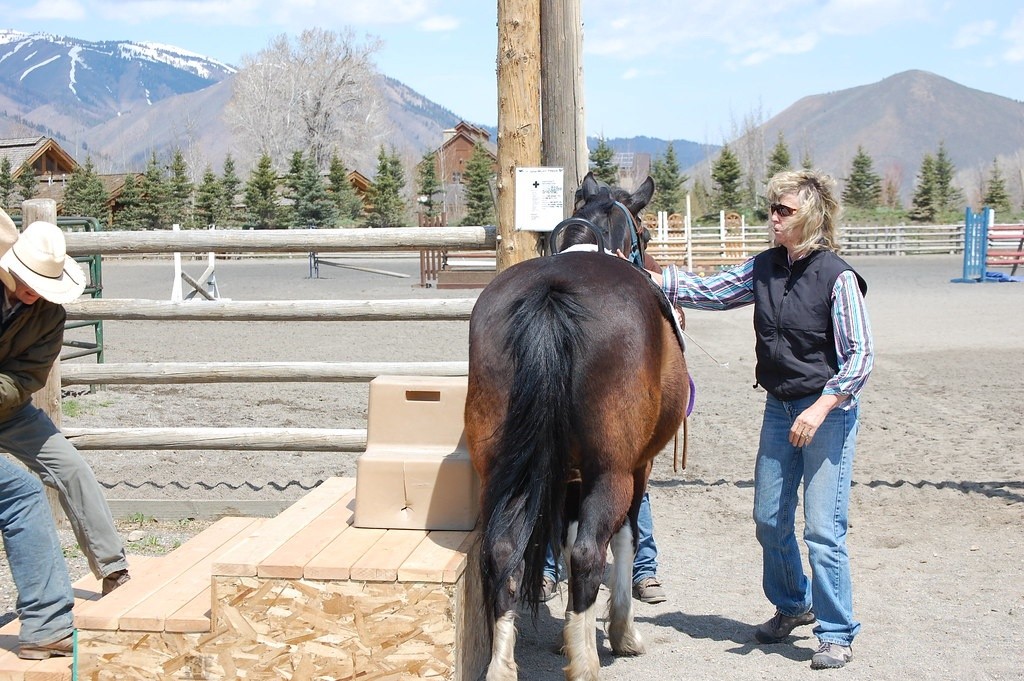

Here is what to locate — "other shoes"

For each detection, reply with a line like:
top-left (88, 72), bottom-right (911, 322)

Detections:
top-left (634, 578), bottom-right (667, 603)
top-left (524, 573), bottom-right (569, 601)
top-left (18, 631), bottom-right (76, 660)
top-left (101, 572), bottom-right (131, 597)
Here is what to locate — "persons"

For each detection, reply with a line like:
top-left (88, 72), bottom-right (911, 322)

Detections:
top-left (0, 209), bottom-right (74, 660)
top-left (0, 220), bottom-right (133, 597)
top-left (617, 168), bottom-right (872, 669)
top-left (540, 490), bottom-right (669, 603)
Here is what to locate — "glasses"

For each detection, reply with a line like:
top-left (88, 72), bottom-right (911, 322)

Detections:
top-left (770, 204), bottom-right (800, 217)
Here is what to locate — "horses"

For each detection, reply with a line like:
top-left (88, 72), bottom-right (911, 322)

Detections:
top-left (464, 170), bottom-right (689, 681)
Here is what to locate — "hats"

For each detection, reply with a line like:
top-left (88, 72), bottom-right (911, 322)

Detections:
top-left (5, 220), bottom-right (87, 304)
top-left (0, 207), bottom-right (19, 293)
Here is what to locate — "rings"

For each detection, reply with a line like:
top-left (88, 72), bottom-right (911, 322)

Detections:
top-left (801, 435), bottom-right (808, 439)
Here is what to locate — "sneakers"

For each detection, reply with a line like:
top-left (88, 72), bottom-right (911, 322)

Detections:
top-left (812, 641), bottom-right (854, 669)
top-left (755, 607), bottom-right (816, 644)
